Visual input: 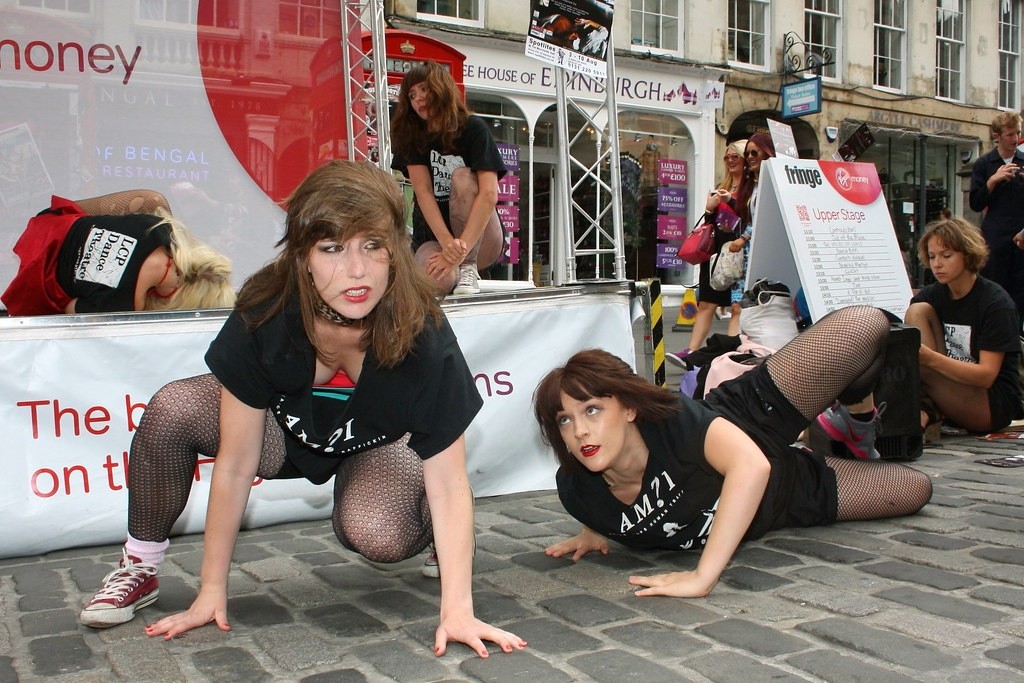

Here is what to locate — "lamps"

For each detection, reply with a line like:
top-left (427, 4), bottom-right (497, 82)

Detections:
top-left (956, 162), bottom-right (974, 192)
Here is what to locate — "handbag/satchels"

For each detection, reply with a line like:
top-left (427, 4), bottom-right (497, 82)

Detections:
top-left (740, 276), bottom-right (799, 351)
top-left (708, 241), bottom-right (744, 291)
top-left (678, 211), bottom-right (715, 265)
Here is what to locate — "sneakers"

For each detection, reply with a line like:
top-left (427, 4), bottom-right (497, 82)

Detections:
top-left (817, 398), bottom-right (887, 460)
top-left (422, 485), bottom-right (476, 577)
top-left (452, 263), bottom-right (481, 295)
top-left (79, 548), bottom-right (160, 628)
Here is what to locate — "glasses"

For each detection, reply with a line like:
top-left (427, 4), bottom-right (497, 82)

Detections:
top-left (724, 154), bottom-right (741, 161)
top-left (744, 149), bottom-right (763, 158)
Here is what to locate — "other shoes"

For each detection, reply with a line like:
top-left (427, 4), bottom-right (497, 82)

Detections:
top-left (665, 349), bottom-right (694, 371)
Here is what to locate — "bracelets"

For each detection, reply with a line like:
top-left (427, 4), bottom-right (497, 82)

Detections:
top-left (740, 235), bottom-right (750, 243)
top-left (706, 210), bottom-right (711, 213)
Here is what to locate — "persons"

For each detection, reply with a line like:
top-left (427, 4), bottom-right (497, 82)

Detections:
top-left (1, 187), bottom-right (239, 316)
top-left (530, 303), bottom-right (933, 598)
top-left (666, 132), bottom-right (776, 368)
top-left (391, 68), bottom-right (507, 296)
top-left (540, 14), bottom-right (607, 59)
top-left (968, 114), bottom-right (1024, 335)
top-left (78, 163), bottom-right (528, 657)
top-left (904, 217), bottom-right (1022, 442)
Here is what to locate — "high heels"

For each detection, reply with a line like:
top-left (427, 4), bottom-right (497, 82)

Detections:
top-left (920, 410), bottom-right (944, 444)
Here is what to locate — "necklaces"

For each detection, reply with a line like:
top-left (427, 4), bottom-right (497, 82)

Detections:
top-left (316, 304), bottom-right (368, 327)
top-left (154, 257), bottom-right (171, 287)
top-left (729, 184), bottom-right (738, 192)
